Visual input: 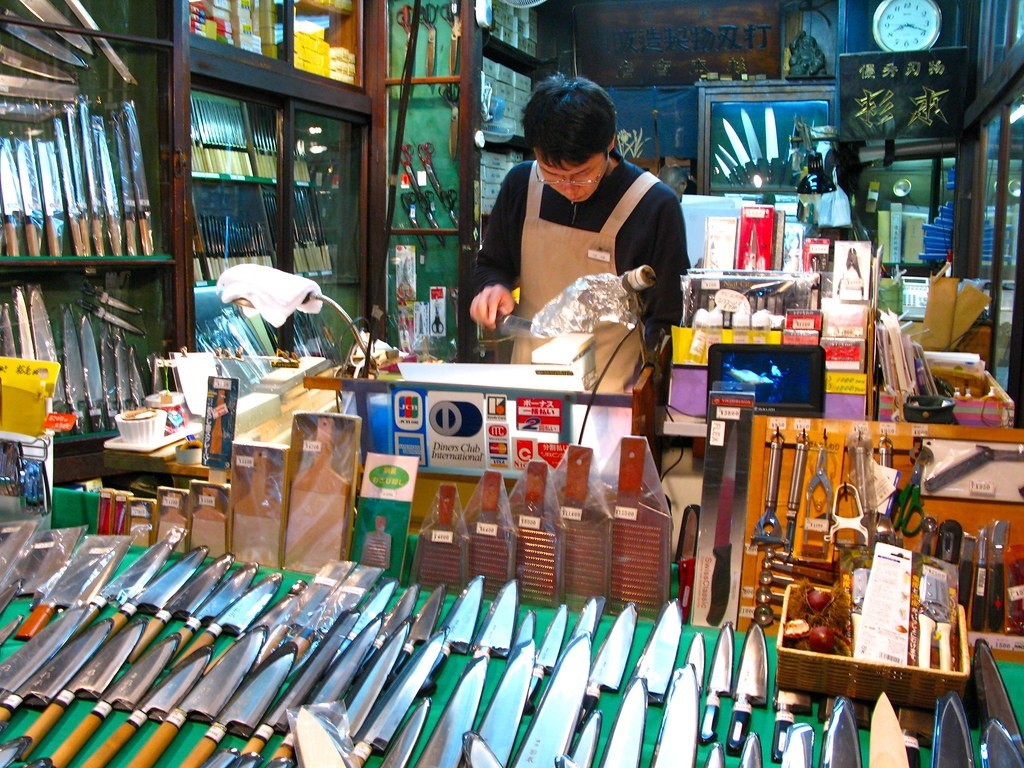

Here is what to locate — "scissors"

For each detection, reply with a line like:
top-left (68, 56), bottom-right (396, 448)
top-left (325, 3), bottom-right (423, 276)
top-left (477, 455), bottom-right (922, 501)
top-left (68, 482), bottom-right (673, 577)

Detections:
top-left (395, 3), bottom-right (437, 85)
top-left (432, 295), bottom-right (444, 334)
top-left (438, 82), bottom-right (460, 164)
top-left (440, 0), bottom-right (462, 77)
top-left (395, 256), bottom-right (414, 301)
top-left (398, 142), bottom-right (460, 251)
top-left (413, 313), bottom-right (429, 352)
top-left (888, 448), bottom-right (925, 537)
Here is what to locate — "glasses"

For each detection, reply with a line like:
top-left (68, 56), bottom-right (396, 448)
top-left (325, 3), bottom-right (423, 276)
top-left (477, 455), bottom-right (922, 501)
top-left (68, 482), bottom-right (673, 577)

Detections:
top-left (535, 153), bottom-right (609, 187)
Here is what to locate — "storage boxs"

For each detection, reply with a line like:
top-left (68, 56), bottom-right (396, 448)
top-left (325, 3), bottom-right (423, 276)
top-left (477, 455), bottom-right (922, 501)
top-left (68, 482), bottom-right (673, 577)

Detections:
top-left (486, 167), bottom-right (507, 184)
top-left (782, 332), bottom-right (818, 347)
top-left (495, 63), bottom-right (514, 85)
top-left (821, 338), bottom-right (865, 373)
top-left (514, 88), bottom-right (530, 106)
top-left (482, 182), bottom-right (502, 198)
top-left (500, 99), bottom-right (515, 118)
top-left (294, 20), bottom-right (356, 85)
top-left (514, 33), bottom-right (525, 52)
top-left (484, 75), bottom-right (495, 96)
top-left (482, 56), bottom-right (497, 77)
top-left (485, 154), bottom-right (507, 168)
top-left (481, 199), bottom-right (499, 215)
top-left (515, 105), bottom-right (525, 122)
top-left (512, 71), bottom-right (532, 92)
top-left (495, 80), bottom-right (514, 102)
top-left (494, 1), bottom-right (538, 43)
top-left (785, 309), bottom-right (822, 331)
top-left (526, 39), bottom-right (539, 57)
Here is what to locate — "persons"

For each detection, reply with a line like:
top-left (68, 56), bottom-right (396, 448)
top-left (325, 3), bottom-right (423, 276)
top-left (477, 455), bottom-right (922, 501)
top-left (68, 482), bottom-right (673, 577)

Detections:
top-left (470, 78), bottom-right (692, 437)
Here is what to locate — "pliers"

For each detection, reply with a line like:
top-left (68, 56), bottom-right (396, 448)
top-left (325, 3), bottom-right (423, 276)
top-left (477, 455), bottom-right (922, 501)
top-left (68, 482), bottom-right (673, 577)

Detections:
top-left (802, 446), bottom-right (834, 559)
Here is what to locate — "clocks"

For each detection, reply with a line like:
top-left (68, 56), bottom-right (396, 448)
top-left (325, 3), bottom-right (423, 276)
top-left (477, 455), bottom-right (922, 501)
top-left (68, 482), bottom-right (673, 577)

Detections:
top-left (872, 0), bottom-right (942, 52)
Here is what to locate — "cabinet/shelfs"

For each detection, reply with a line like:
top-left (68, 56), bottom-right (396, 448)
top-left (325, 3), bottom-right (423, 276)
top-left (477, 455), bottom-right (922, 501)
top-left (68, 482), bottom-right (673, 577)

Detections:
top-left (181, 0), bottom-right (378, 353)
top-left (0, 0), bottom-right (178, 485)
top-left (379, 0), bottom-right (485, 361)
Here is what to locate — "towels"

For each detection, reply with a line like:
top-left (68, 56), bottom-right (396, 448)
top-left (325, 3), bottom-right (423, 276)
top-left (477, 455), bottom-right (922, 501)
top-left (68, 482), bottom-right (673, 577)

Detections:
top-left (214, 262), bottom-right (323, 330)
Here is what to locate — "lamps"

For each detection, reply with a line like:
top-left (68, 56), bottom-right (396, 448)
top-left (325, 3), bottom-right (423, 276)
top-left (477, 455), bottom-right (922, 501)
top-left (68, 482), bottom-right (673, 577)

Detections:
top-left (754, 172), bottom-right (774, 205)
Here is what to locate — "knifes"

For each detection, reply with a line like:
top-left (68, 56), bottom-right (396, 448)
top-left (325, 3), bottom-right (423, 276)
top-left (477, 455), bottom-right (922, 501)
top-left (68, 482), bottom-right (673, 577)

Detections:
top-left (1, 0), bottom-right (1024, 768)
